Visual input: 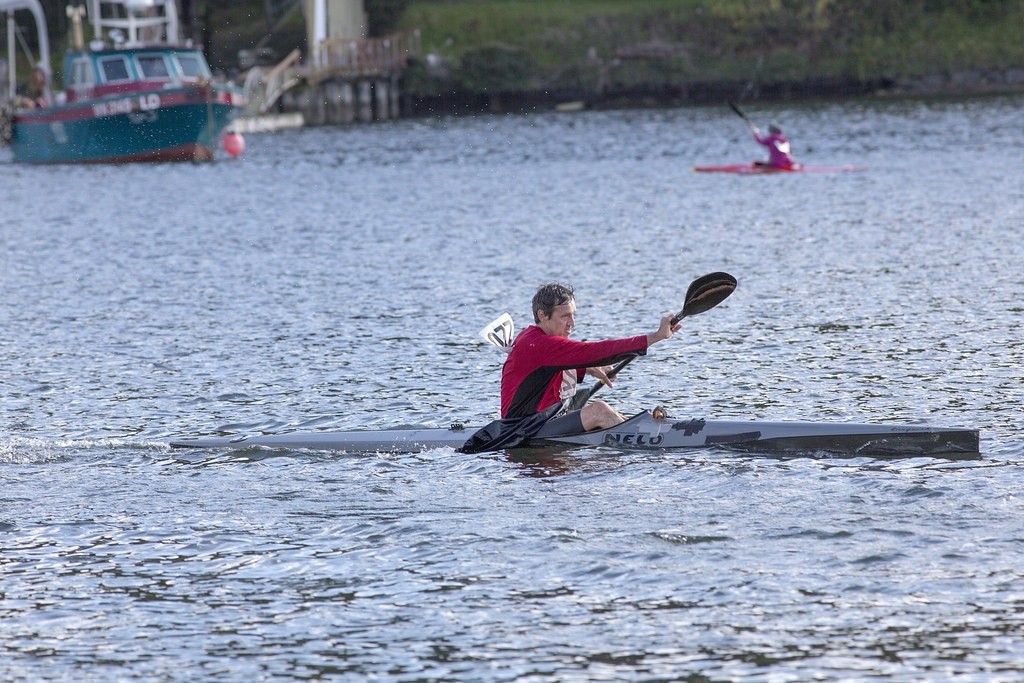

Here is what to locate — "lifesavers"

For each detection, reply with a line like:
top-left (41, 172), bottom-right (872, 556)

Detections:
top-left (32, 67), bottom-right (47, 85)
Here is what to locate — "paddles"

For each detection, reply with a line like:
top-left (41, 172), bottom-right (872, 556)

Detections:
top-left (729, 101), bottom-right (757, 130)
top-left (570, 271), bottom-right (738, 412)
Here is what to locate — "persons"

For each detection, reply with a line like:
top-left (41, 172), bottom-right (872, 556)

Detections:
top-left (752, 124), bottom-right (794, 169)
top-left (20, 67), bottom-right (46, 109)
top-left (500, 284), bottom-right (682, 435)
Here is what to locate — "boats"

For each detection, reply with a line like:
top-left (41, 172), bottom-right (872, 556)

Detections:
top-left (696, 164), bottom-right (865, 175)
top-left (0, 0), bottom-right (249, 164)
top-left (168, 406), bottom-right (982, 454)
